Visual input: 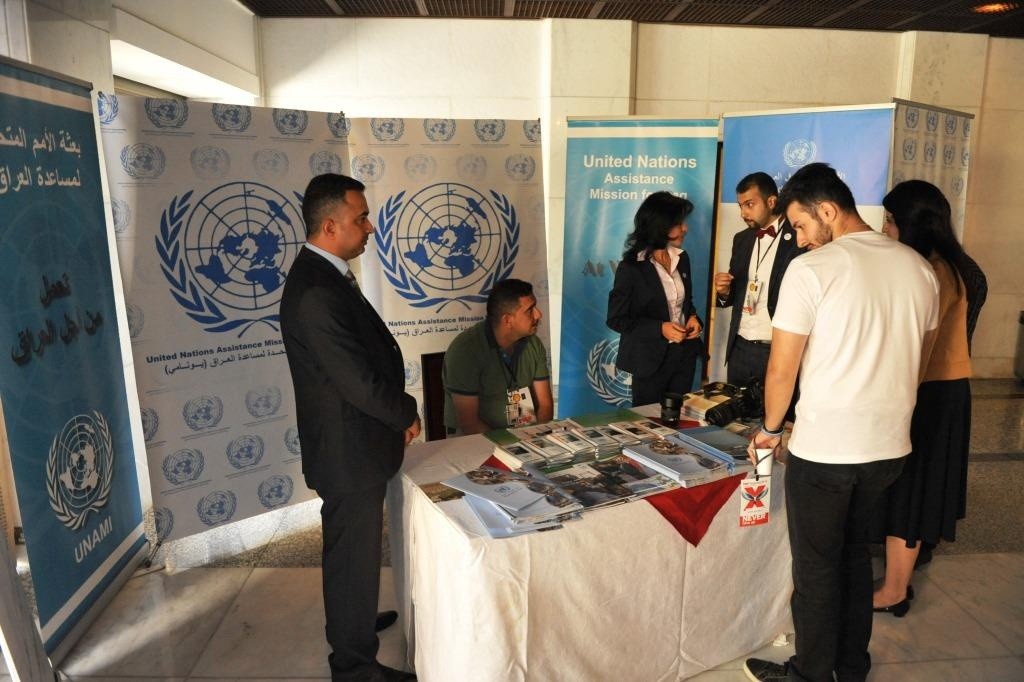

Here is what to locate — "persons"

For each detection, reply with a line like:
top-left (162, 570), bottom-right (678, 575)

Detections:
top-left (716, 171), bottom-right (809, 423)
top-left (871, 180), bottom-right (989, 617)
top-left (443, 279), bottom-right (554, 438)
top-left (279, 173), bottom-right (422, 682)
top-left (742, 163), bottom-right (940, 682)
top-left (606, 191), bottom-right (711, 408)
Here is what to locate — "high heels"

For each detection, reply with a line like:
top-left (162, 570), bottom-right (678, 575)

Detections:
top-left (906, 585), bottom-right (914, 599)
top-left (873, 598), bottom-right (910, 617)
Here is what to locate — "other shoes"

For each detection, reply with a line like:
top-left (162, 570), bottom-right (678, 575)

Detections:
top-left (833, 670), bottom-right (866, 682)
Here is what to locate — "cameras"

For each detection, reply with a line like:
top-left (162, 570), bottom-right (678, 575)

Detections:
top-left (702, 378), bottom-right (764, 427)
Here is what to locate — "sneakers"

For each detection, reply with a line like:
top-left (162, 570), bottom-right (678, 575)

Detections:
top-left (743, 657), bottom-right (808, 682)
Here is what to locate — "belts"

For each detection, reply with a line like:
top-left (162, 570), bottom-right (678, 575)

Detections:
top-left (738, 335), bottom-right (771, 345)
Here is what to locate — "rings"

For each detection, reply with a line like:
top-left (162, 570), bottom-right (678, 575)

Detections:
top-left (699, 329), bottom-right (703, 335)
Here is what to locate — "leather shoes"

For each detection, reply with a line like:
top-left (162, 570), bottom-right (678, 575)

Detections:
top-left (378, 664), bottom-right (417, 682)
top-left (374, 610), bottom-right (398, 633)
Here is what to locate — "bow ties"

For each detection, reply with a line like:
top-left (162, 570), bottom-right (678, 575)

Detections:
top-left (755, 214), bottom-right (786, 239)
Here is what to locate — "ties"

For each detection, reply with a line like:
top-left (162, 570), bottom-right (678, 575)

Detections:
top-left (345, 268), bottom-right (363, 302)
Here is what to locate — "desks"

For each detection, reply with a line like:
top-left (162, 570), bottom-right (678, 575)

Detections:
top-left (388, 400), bottom-right (803, 682)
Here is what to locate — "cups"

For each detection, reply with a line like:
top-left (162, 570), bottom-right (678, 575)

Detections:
top-left (753, 448), bottom-right (774, 475)
top-left (658, 392), bottom-right (683, 428)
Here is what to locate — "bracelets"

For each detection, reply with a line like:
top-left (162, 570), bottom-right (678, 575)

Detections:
top-left (761, 423), bottom-right (785, 437)
